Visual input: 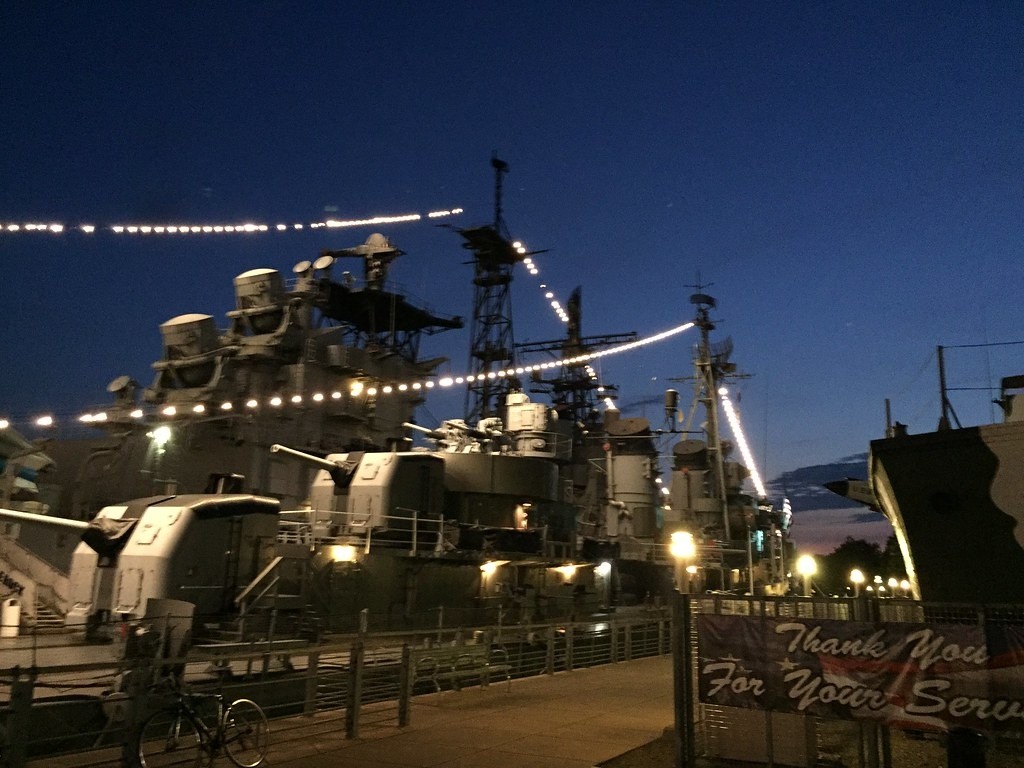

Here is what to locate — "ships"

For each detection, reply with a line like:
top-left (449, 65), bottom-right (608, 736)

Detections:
top-left (0, 151), bottom-right (804, 744)
top-left (868, 341), bottom-right (1024, 605)
